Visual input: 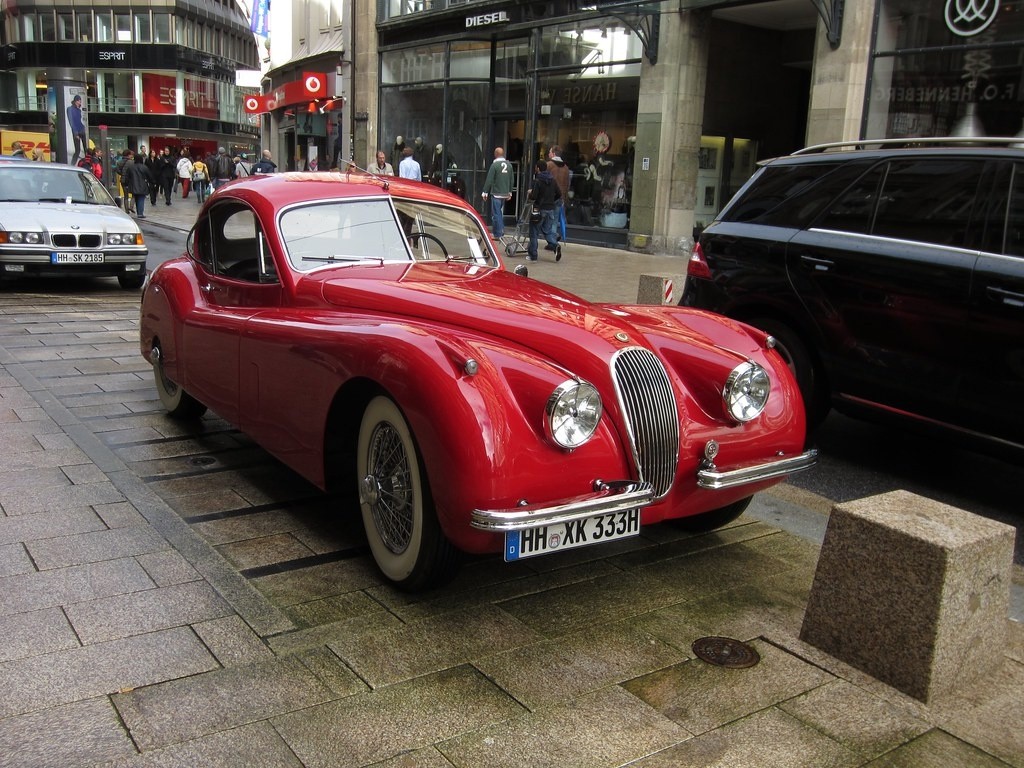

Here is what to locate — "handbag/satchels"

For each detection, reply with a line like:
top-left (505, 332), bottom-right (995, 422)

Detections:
top-left (205, 181), bottom-right (215, 195)
top-left (192, 171), bottom-right (206, 182)
top-left (173, 177), bottom-right (179, 193)
top-left (531, 211), bottom-right (541, 223)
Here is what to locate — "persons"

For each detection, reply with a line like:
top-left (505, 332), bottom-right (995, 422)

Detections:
top-left (429, 144), bottom-right (457, 186)
top-left (11, 141), bottom-right (27, 158)
top-left (66, 95), bottom-right (87, 165)
top-left (76, 145), bottom-right (278, 218)
top-left (600, 168), bottom-right (625, 207)
top-left (546, 145), bottom-right (570, 249)
top-left (366, 151), bottom-right (395, 176)
top-left (525, 160), bottom-right (562, 263)
top-left (398, 146), bottom-right (422, 181)
top-left (481, 147), bottom-right (514, 241)
top-left (301, 111), bottom-right (342, 169)
top-left (389, 135), bottom-right (406, 177)
top-left (32, 148), bottom-right (45, 161)
top-left (412, 137), bottom-right (432, 176)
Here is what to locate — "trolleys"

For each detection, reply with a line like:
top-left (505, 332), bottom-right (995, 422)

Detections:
top-left (499, 191), bottom-right (536, 258)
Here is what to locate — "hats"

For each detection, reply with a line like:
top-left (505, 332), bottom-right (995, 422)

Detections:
top-left (110, 148), bottom-right (114, 151)
top-left (241, 153), bottom-right (248, 159)
top-left (74, 95), bottom-right (81, 100)
top-left (218, 147), bottom-right (225, 153)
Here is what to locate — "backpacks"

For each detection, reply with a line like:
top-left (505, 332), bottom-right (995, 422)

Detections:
top-left (81, 158), bottom-right (94, 173)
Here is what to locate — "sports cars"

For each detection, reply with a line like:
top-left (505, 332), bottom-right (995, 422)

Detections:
top-left (139, 170), bottom-right (820, 597)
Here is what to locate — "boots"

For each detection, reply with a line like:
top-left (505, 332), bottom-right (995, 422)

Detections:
top-left (124, 199), bottom-right (130, 214)
top-left (129, 198), bottom-right (136, 213)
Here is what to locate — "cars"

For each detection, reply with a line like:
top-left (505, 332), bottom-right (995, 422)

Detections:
top-left (0, 159), bottom-right (148, 292)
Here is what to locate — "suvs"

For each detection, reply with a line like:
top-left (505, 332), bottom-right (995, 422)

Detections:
top-left (676, 138), bottom-right (1024, 476)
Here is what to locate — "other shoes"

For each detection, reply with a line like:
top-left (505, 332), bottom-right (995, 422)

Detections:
top-left (137, 215), bottom-right (146, 218)
top-left (525, 256), bottom-right (537, 263)
top-left (198, 200), bottom-right (201, 203)
top-left (491, 236), bottom-right (501, 241)
top-left (202, 200), bottom-right (205, 203)
top-left (556, 233), bottom-right (561, 241)
top-left (544, 243), bottom-right (554, 250)
top-left (553, 245), bottom-right (561, 262)
